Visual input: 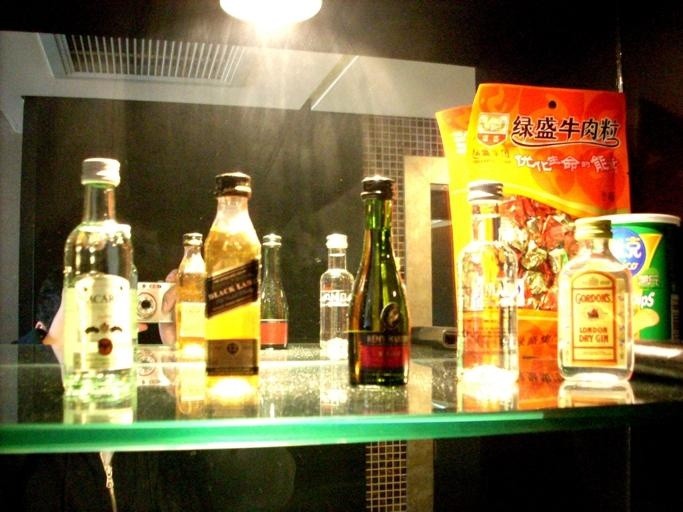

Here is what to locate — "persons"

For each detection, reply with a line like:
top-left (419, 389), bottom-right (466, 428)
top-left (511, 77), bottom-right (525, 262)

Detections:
top-left (1, 267), bottom-right (298, 511)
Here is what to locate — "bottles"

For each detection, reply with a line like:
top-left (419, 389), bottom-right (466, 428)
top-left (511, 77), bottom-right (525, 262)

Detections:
top-left (60, 157), bottom-right (137, 399)
top-left (257, 234), bottom-right (290, 351)
top-left (556, 219), bottom-right (636, 386)
top-left (170, 232), bottom-right (209, 359)
top-left (456, 181), bottom-right (519, 381)
top-left (200, 170), bottom-right (262, 397)
top-left (318, 232), bottom-right (356, 351)
top-left (349, 176), bottom-right (409, 391)
